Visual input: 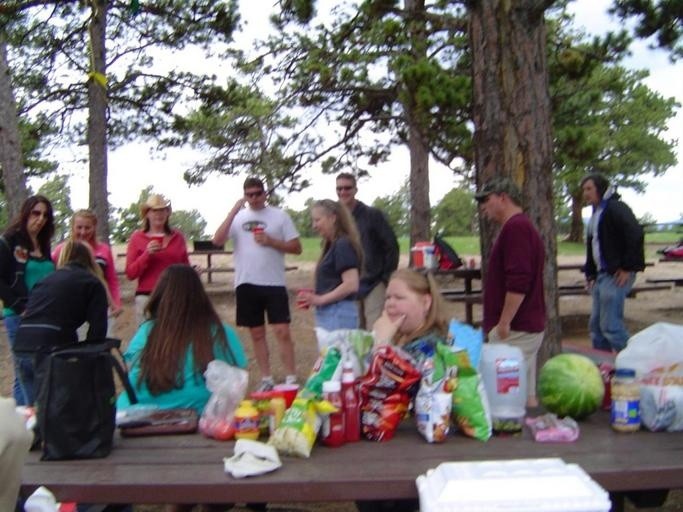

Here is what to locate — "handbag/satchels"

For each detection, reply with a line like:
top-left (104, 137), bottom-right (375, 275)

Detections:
top-left (432, 232), bottom-right (462, 275)
top-left (28, 337), bottom-right (138, 462)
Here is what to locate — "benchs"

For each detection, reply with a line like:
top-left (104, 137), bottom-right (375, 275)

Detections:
top-left (440, 281), bottom-right (669, 304)
top-left (114, 266), bottom-right (235, 277)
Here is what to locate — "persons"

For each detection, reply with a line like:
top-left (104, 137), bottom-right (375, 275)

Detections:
top-left (0, 194), bottom-right (56, 511)
top-left (51, 208), bottom-right (125, 341)
top-left (210, 177), bottom-right (303, 394)
top-left (472, 174), bottom-right (548, 411)
top-left (101, 262), bottom-right (249, 512)
top-left (294, 197), bottom-right (371, 383)
top-left (9, 236), bottom-right (117, 413)
top-left (127, 192), bottom-right (190, 329)
top-left (351, 266), bottom-right (454, 512)
top-left (577, 173), bottom-right (646, 353)
top-left (322, 172), bottom-right (398, 331)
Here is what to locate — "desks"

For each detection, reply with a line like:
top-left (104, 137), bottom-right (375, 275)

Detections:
top-left (111, 248), bottom-right (299, 284)
top-left (19, 415), bottom-right (682, 512)
top-left (412, 262), bottom-right (674, 331)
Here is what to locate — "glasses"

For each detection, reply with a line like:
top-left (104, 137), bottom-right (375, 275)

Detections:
top-left (337, 187), bottom-right (351, 191)
top-left (245, 189), bottom-right (264, 198)
top-left (30, 209), bottom-right (49, 217)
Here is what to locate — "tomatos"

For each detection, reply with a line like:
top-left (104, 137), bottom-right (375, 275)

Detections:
top-left (201, 415), bottom-right (235, 440)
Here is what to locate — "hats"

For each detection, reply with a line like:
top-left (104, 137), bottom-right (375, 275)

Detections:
top-left (475, 176), bottom-right (522, 205)
top-left (139, 194), bottom-right (172, 221)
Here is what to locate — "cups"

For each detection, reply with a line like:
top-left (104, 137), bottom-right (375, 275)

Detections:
top-left (273, 383), bottom-right (300, 407)
top-left (148, 232), bottom-right (165, 253)
top-left (252, 227), bottom-right (265, 242)
top-left (293, 286), bottom-right (314, 310)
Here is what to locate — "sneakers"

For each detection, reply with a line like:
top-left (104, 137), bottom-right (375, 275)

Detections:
top-left (256, 382), bottom-right (274, 392)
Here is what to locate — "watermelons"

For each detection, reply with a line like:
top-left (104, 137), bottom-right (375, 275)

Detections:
top-left (534, 351), bottom-right (605, 421)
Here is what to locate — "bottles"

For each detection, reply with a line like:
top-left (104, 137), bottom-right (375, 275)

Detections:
top-left (232, 400), bottom-right (259, 440)
top-left (320, 361), bottom-right (361, 446)
top-left (610, 369), bottom-right (640, 432)
top-left (598, 363), bottom-right (614, 408)
